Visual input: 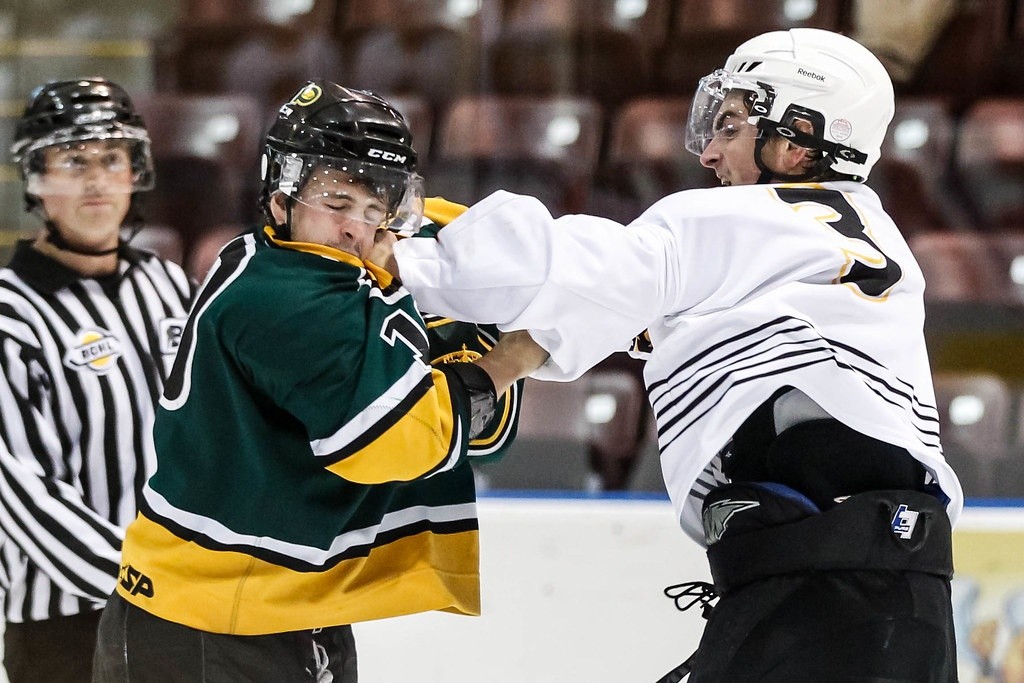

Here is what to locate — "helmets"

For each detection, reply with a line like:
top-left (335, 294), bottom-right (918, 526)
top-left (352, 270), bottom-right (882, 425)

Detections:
top-left (15, 78), bottom-right (150, 155)
top-left (265, 77), bottom-right (418, 183)
top-left (721, 26), bottom-right (895, 181)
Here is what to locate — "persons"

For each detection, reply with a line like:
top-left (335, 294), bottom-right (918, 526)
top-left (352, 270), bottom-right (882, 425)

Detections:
top-left (368, 28), bottom-right (965, 683)
top-left (93, 77), bottom-right (653, 683)
top-left (0, 78), bottom-right (202, 683)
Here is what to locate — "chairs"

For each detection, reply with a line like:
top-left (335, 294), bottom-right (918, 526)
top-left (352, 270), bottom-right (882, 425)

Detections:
top-left (127, 0), bottom-right (1024, 501)
